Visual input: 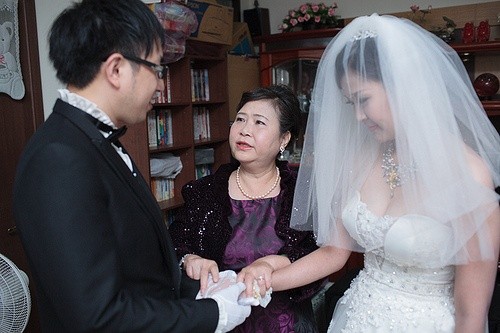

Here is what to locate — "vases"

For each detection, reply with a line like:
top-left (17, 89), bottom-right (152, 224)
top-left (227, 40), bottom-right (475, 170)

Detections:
top-left (303, 21), bottom-right (323, 30)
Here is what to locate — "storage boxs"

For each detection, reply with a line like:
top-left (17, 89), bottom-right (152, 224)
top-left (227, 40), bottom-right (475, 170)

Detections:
top-left (187, 0), bottom-right (259, 121)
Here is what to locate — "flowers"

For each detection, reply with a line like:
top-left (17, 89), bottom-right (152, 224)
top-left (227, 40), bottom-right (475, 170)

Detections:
top-left (280, 3), bottom-right (341, 30)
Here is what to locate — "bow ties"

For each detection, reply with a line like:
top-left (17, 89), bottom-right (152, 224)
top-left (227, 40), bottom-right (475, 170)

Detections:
top-left (66, 102), bottom-right (128, 143)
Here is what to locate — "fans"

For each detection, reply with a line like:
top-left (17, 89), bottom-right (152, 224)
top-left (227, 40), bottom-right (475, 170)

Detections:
top-left (0, 254), bottom-right (31, 333)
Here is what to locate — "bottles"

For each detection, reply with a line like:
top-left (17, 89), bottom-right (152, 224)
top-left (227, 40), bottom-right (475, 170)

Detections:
top-left (463, 22), bottom-right (475, 44)
top-left (477, 21), bottom-right (490, 42)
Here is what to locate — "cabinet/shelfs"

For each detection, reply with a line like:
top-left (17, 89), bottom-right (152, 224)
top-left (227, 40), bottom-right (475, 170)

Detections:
top-left (258, 48), bottom-right (325, 172)
top-left (451, 42), bottom-right (500, 115)
top-left (120, 39), bottom-right (231, 213)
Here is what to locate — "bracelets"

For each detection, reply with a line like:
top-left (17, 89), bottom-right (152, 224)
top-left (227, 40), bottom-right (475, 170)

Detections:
top-left (178, 253), bottom-right (196, 269)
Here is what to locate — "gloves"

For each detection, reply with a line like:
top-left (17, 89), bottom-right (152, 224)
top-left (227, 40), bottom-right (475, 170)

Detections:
top-left (196, 269), bottom-right (251, 333)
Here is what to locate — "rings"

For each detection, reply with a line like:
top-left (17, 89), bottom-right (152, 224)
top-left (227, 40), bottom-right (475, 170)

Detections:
top-left (255, 276), bottom-right (265, 281)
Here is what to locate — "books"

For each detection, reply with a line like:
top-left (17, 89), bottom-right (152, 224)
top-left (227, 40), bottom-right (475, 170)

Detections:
top-left (146, 62), bottom-right (215, 203)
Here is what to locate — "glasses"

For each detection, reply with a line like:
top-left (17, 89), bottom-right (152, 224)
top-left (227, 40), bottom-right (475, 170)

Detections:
top-left (102, 50), bottom-right (168, 79)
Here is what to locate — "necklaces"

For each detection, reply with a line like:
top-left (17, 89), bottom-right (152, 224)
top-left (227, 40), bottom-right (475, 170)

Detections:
top-left (236, 165), bottom-right (280, 200)
top-left (380, 141), bottom-right (434, 199)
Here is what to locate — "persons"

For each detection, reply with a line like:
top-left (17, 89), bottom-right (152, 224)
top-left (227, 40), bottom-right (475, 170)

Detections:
top-left (164, 83), bottom-right (329, 333)
top-left (11, 1), bottom-right (252, 333)
top-left (203, 10), bottom-right (500, 333)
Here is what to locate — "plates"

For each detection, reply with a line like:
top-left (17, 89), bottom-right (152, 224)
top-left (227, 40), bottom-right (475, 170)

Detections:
top-left (473, 73), bottom-right (499, 97)
